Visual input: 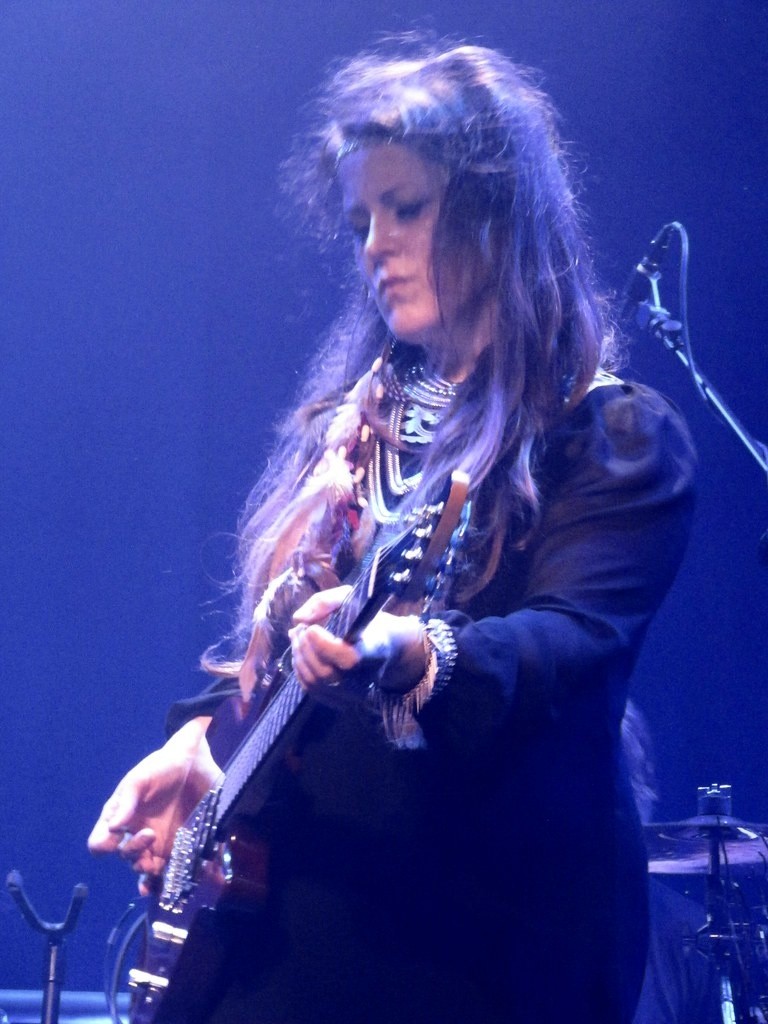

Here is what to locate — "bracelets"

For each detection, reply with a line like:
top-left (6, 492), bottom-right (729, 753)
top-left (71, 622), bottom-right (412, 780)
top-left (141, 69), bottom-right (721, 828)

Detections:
top-left (428, 617), bottom-right (458, 693)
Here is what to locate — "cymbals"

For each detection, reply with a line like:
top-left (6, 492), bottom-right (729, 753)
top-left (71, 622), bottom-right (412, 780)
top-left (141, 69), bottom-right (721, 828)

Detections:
top-left (641, 815), bottom-right (768, 828)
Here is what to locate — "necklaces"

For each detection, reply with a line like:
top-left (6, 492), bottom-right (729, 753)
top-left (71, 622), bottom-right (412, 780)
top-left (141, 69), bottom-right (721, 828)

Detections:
top-left (407, 360), bottom-right (461, 408)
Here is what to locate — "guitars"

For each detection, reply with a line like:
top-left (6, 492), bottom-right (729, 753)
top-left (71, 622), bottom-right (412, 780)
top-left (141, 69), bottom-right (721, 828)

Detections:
top-left (104, 469), bottom-right (471, 1024)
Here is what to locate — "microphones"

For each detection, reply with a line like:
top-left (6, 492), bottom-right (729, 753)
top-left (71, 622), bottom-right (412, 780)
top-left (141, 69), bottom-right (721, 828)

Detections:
top-left (599, 225), bottom-right (674, 367)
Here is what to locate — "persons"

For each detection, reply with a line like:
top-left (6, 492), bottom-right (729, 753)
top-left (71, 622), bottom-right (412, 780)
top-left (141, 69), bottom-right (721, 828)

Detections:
top-left (86, 45), bottom-right (699, 1024)
